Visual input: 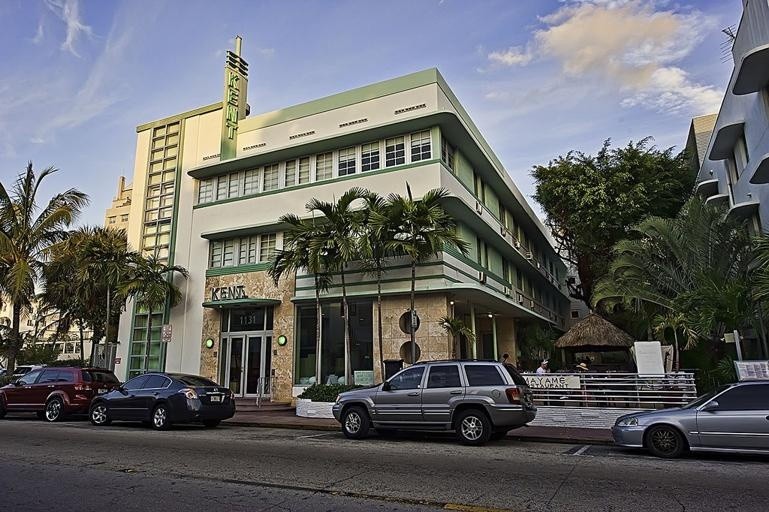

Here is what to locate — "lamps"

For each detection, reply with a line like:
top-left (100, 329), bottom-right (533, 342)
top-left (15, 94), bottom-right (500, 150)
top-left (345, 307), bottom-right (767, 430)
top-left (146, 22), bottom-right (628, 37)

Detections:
top-left (448, 300), bottom-right (455, 305)
top-left (479, 312), bottom-right (492, 318)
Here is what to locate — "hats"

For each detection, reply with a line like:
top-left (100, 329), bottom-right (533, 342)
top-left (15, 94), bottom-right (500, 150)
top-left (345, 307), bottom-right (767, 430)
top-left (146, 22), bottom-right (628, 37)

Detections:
top-left (541, 359), bottom-right (549, 365)
top-left (576, 363), bottom-right (589, 370)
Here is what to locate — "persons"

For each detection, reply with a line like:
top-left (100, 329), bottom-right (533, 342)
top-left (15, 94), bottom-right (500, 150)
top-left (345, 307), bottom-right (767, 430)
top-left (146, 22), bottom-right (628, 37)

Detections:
top-left (570, 354), bottom-right (593, 374)
top-left (535, 358), bottom-right (554, 373)
top-left (502, 353), bottom-right (510, 364)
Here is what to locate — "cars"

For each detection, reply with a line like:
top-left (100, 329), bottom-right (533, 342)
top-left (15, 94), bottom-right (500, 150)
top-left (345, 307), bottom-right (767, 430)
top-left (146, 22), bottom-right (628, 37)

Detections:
top-left (611, 379), bottom-right (769, 458)
top-left (0, 366), bottom-right (236, 430)
top-left (332, 358), bottom-right (537, 447)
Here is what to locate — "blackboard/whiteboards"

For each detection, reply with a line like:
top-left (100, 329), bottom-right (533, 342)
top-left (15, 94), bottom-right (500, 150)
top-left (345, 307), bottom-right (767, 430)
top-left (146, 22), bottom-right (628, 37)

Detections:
top-left (354, 371), bottom-right (374, 385)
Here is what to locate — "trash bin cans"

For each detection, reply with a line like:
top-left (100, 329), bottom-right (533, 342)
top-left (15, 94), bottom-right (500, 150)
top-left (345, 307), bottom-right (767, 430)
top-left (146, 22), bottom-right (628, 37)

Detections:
top-left (382, 359), bottom-right (404, 380)
